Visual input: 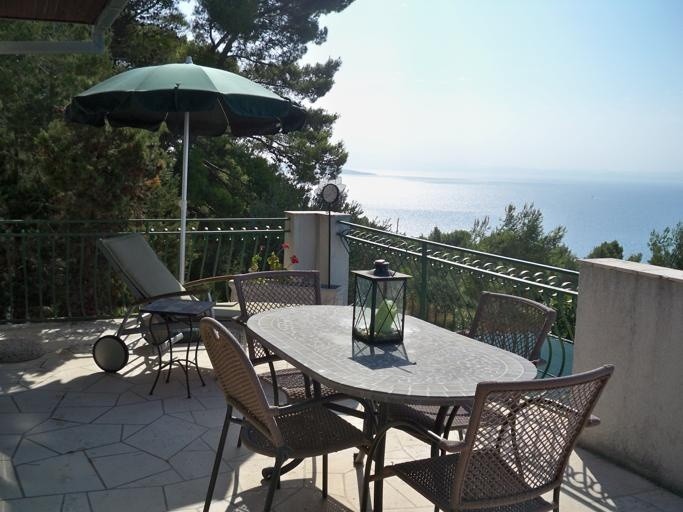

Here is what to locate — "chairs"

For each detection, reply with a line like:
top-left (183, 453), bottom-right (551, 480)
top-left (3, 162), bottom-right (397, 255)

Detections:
top-left (360, 363), bottom-right (615, 511)
top-left (231, 270), bottom-right (376, 500)
top-left (370, 290), bottom-right (558, 510)
top-left (199, 316), bottom-right (381, 512)
top-left (93, 232), bottom-right (255, 374)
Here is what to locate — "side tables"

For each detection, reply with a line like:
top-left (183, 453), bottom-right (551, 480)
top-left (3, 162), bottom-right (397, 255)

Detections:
top-left (138, 299), bottom-right (213, 399)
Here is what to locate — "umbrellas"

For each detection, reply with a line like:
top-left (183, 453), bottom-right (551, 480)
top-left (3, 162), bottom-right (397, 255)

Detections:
top-left (64, 56), bottom-right (306, 284)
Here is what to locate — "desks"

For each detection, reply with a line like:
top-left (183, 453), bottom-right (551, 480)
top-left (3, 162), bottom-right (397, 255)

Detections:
top-left (246, 305), bottom-right (539, 512)
